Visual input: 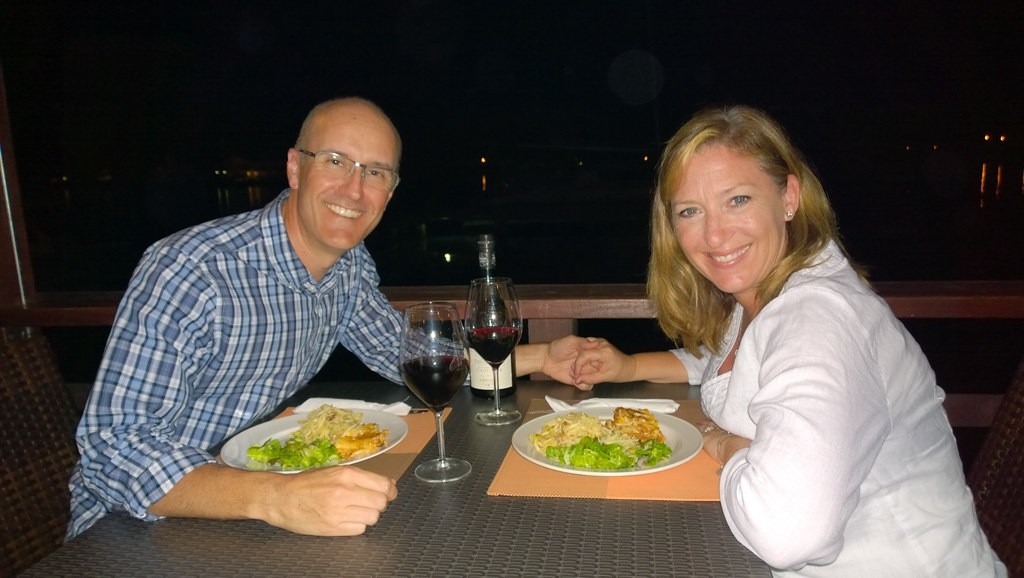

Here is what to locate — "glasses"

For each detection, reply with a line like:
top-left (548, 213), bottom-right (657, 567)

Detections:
top-left (296, 149), bottom-right (400, 194)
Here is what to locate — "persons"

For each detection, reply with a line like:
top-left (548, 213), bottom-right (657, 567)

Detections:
top-left (571, 109), bottom-right (1011, 578)
top-left (62, 96), bottom-right (600, 542)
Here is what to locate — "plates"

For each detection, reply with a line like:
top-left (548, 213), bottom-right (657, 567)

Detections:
top-left (220, 408), bottom-right (408, 475)
top-left (511, 407), bottom-right (704, 477)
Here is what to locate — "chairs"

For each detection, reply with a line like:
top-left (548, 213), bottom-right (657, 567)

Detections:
top-left (968, 354), bottom-right (1024, 578)
top-left (0, 326), bottom-right (78, 578)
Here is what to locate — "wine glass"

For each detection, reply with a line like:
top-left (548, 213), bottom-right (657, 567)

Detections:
top-left (464, 277), bottom-right (524, 426)
top-left (398, 302), bottom-right (472, 483)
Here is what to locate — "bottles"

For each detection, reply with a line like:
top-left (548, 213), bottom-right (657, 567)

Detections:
top-left (468, 234), bottom-right (518, 400)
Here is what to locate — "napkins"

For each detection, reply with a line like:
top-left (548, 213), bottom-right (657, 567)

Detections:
top-left (291, 397), bottom-right (412, 417)
top-left (543, 395), bottom-right (681, 413)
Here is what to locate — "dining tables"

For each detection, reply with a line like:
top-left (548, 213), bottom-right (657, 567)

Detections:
top-left (22, 380), bottom-right (773, 578)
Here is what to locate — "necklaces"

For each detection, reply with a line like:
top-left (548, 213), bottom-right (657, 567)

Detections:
top-left (735, 326), bottom-right (742, 355)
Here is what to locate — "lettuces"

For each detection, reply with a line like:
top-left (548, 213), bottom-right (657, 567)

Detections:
top-left (246, 437), bottom-right (337, 471)
top-left (546, 436), bottom-right (674, 469)
